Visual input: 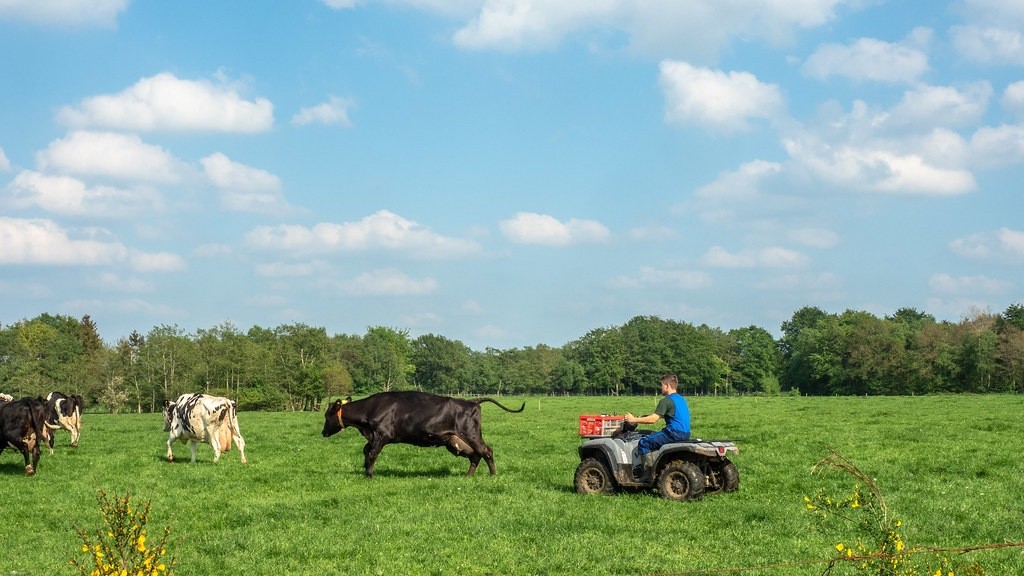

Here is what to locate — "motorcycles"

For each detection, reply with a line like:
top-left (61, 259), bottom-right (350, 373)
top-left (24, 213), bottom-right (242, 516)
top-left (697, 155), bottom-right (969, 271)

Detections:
top-left (574, 413), bottom-right (741, 502)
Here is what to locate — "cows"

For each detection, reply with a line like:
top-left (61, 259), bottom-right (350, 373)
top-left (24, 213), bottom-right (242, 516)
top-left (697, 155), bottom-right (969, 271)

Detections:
top-left (321, 390), bottom-right (526, 476)
top-left (161, 392), bottom-right (246, 464)
top-left (45, 391), bottom-right (85, 448)
top-left (0, 393), bottom-right (46, 475)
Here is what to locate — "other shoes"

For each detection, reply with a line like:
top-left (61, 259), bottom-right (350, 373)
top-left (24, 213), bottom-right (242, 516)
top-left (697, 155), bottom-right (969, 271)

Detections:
top-left (634, 464), bottom-right (653, 483)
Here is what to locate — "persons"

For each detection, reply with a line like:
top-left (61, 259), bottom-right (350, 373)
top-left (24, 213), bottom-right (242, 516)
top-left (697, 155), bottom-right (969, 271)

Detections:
top-left (624, 374), bottom-right (690, 482)
